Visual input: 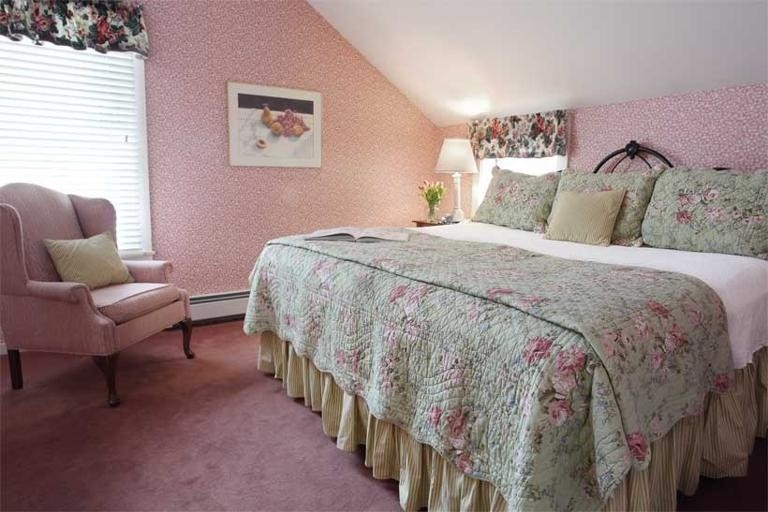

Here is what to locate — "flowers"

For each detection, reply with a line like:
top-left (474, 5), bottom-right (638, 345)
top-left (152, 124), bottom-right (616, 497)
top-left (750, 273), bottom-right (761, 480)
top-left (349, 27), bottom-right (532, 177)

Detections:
top-left (419, 181), bottom-right (446, 208)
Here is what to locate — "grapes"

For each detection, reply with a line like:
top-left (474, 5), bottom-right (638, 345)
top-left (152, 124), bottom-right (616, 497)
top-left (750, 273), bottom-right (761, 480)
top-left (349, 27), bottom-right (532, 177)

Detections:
top-left (275, 109), bottom-right (294, 136)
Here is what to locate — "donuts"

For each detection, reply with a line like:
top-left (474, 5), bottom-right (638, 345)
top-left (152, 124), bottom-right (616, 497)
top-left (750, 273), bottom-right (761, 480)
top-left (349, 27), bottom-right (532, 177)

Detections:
top-left (257, 138), bottom-right (268, 148)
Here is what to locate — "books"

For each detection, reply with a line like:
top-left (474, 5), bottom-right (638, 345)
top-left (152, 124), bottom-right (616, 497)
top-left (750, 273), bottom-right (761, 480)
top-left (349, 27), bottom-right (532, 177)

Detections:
top-left (302, 225), bottom-right (410, 246)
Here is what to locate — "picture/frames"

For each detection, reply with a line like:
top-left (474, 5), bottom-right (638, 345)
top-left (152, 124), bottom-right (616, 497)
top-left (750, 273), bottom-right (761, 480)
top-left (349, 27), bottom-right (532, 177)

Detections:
top-left (227, 81), bottom-right (322, 169)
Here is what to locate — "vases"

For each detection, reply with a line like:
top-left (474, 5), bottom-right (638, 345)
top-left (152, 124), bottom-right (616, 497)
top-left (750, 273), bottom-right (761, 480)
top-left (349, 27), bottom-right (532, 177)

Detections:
top-left (427, 209), bottom-right (437, 219)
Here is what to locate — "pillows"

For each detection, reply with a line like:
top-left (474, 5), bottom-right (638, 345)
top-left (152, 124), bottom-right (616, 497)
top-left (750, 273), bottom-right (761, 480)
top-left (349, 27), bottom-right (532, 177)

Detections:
top-left (45, 232), bottom-right (136, 291)
top-left (546, 164), bottom-right (665, 247)
top-left (471, 165), bottom-right (561, 233)
top-left (543, 189), bottom-right (626, 247)
top-left (642, 168), bottom-right (768, 260)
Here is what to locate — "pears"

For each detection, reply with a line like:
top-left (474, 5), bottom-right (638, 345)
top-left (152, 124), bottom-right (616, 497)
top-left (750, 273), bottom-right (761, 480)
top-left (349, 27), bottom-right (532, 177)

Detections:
top-left (261, 104), bottom-right (273, 125)
top-left (269, 122), bottom-right (283, 136)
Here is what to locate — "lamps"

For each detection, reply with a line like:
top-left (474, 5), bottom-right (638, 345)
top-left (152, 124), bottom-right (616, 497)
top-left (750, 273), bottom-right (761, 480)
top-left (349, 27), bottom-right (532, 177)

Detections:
top-left (434, 139), bottom-right (479, 223)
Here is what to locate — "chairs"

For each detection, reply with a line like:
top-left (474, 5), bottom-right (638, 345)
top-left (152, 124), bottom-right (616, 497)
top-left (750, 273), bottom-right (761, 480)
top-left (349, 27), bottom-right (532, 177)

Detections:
top-left (1, 184), bottom-right (195, 406)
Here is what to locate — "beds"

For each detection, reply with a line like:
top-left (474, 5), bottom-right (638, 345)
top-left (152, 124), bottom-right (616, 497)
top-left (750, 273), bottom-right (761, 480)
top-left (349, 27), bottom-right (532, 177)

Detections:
top-left (243, 140), bottom-right (768, 512)
